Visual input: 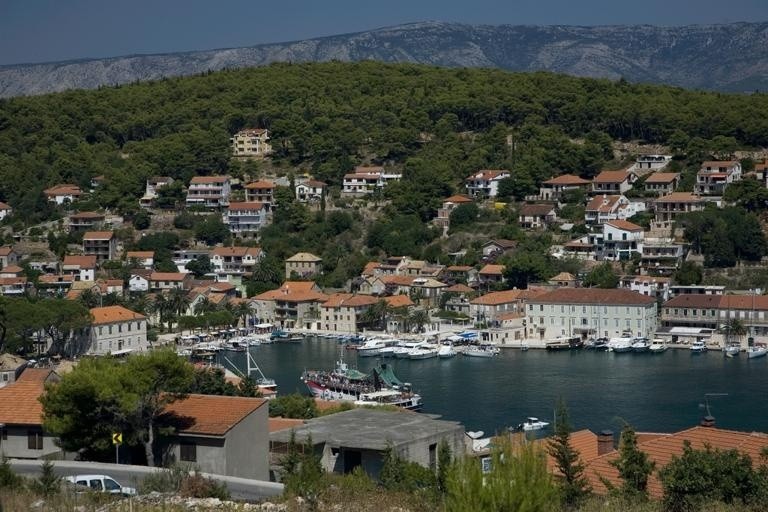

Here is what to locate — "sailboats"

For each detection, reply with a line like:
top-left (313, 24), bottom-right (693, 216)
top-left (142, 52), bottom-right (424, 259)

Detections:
top-left (247, 340), bottom-right (277, 390)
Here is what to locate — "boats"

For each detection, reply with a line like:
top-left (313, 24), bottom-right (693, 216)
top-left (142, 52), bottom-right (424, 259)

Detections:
top-left (545, 337), bottom-right (668, 353)
top-left (301, 345), bottom-right (424, 412)
top-left (746, 345), bottom-right (767, 359)
top-left (357, 333), bottom-right (500, 358)
top-left (722, 341), bottom-right (740, 355)
top-left (689, 341), bottom-right (707, 352)
top-left (307, 333), bottom-right (346, 339)
top-left (179, 333), bottom-right (303, 360)
top-left (519, 417), bottom-right (550, 431)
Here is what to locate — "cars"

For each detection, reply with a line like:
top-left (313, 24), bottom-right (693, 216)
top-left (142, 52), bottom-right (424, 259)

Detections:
top-left (61, 474), bottom-right (135, 498)
